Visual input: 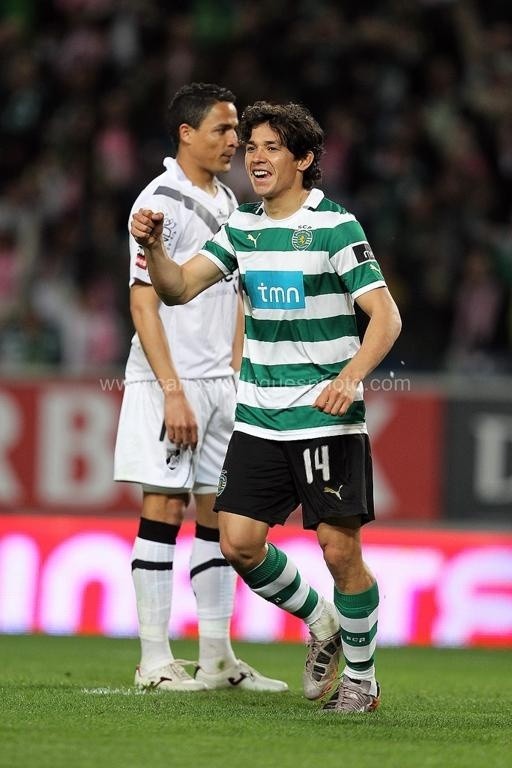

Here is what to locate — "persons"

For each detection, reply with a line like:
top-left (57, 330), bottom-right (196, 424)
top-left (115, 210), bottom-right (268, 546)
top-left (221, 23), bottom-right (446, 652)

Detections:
top-left (114, 84), bottom-right (291, 691)
top-left (129, 98), bottom-right (408, 712)
top-left (2, 1), bottom-right (511, 385)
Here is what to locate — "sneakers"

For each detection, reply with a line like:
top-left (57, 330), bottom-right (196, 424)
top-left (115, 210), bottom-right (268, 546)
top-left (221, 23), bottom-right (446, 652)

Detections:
top-left (135, 659), bottom-right (289, 692)
top-left (303, 630), bottom-right (380, 714)
